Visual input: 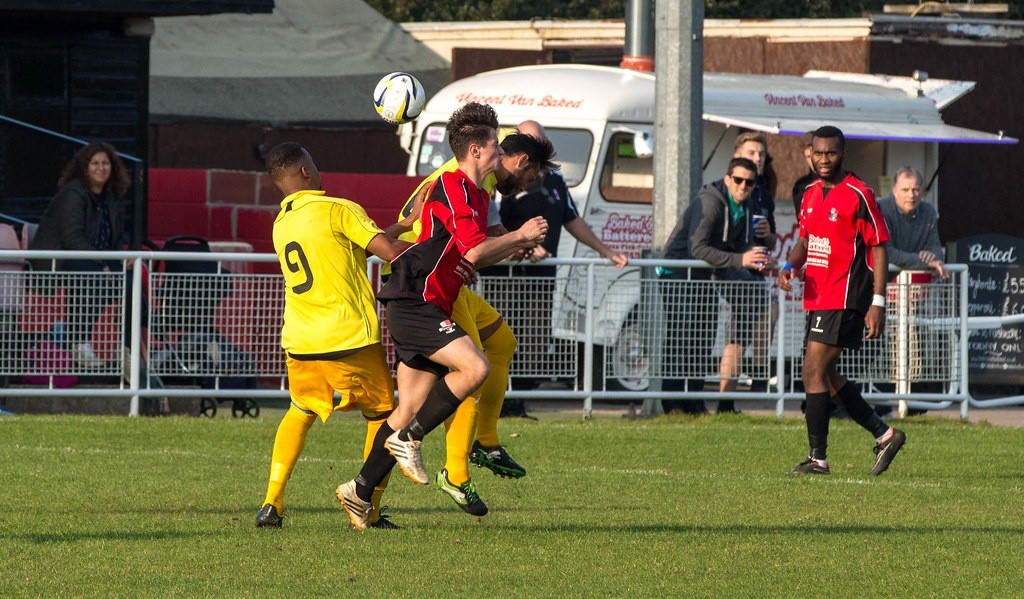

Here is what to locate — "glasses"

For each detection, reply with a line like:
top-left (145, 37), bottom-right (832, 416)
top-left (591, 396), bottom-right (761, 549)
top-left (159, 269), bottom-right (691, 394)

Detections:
top-left (732, 176), bottom-right (755, 187)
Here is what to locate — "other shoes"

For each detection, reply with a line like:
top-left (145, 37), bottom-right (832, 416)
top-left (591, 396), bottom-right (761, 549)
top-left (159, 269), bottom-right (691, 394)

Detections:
top-left (71, 343), bottom-right (103, 367)
top-left (667, 409), bottom-right (711, 415)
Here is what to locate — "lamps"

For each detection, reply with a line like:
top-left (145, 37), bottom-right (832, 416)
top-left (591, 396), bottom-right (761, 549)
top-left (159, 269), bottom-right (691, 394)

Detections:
top-left (124, 15), bottom-right (155, 36)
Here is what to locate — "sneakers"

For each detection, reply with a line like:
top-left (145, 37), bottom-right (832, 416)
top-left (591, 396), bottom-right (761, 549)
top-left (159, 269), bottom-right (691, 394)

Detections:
top-left (384, 429), bottom-right (429, 485)
top-left (336, 479), bottom-right (375, 532)
top-left (436, 469), bottom-right (488, 517)
top-left (469, 440), bottom-right (526, 479)
top-left (255, 504), bottom-right (284, 529)
top-left (349, 517), bottom-right (405, 530)
top-left (785, 456), bottom-right (830, 475)
top-left (867, 428), bottom-right (906, 476)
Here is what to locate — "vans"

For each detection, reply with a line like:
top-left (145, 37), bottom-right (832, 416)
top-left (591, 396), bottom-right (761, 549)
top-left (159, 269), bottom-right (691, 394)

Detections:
top-left (397, 70), bottom-right (1020, 405)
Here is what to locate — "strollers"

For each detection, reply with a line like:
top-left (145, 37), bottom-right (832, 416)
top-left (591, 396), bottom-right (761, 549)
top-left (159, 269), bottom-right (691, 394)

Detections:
top-left (115, 237), bottom-right (261, 418)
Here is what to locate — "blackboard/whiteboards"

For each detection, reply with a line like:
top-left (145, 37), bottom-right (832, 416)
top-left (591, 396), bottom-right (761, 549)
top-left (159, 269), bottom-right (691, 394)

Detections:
top-left (947, 233), bottom-right (1024, 385)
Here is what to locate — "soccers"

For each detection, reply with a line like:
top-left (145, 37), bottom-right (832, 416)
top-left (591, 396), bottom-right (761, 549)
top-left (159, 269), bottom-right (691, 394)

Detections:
top-left (372, 71), bottom-right (426, 125)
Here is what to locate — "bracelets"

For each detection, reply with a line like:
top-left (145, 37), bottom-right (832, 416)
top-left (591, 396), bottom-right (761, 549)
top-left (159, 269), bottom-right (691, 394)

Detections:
top-left (871, 294), bottom-right (885, 309)
top-left (781, 263), bottom-right (794, 270)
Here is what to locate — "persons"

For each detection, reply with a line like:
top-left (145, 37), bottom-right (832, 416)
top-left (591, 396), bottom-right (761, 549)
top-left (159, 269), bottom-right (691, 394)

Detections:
top-left (336, 99), bottom-right (547, 527)
top-left (791, 140), bottom-right (816, 220)
top-left (255, 141), bottom-right (432, 529)
top-left (27, 142), bottom-right (147, 387)
top-left (714, 131), bottom-right (778, 412)
top-left (873, 167), bottom-right (949, 418)
top-left (381, 135), bottom-right (551, 521)
top-left (478, 119), bottom-right (627, 421)
top-left (653, 157), bottom-right (771, 420)
top-left (777, 126), bottom-right (905, 476)
